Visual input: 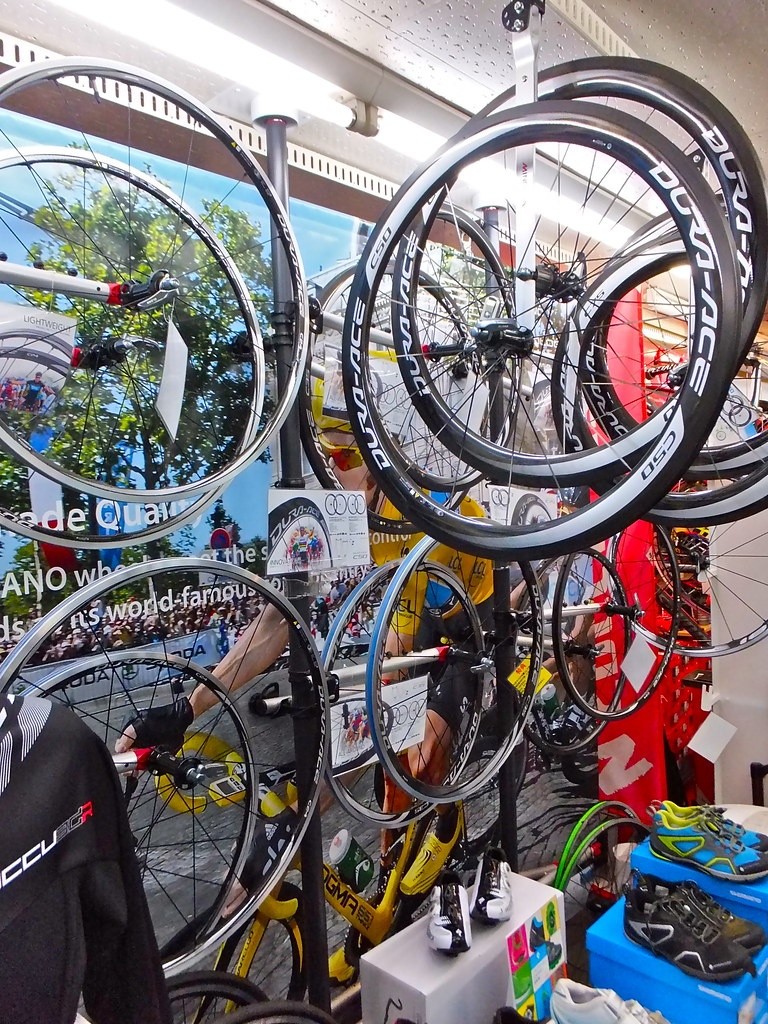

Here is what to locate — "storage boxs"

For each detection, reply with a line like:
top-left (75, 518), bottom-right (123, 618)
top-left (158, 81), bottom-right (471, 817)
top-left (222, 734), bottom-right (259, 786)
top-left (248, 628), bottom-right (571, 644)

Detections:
top-left (586, 895), bottom-right (768, 1024)
top-left (359, 872), bottom-right (569, 1024)
top-left (630, 834), bottom-right (768, 944)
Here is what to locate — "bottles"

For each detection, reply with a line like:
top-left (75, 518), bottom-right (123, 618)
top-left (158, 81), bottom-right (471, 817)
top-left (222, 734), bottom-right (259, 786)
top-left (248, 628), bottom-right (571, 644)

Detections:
top-left (329, 828), bottom-right (372, 895)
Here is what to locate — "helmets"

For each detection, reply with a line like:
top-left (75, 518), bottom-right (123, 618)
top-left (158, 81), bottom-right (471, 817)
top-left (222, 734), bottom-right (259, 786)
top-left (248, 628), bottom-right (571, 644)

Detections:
top-left (556, 485), bottom-right (589, 508)
top-left (311, 350), bottom-right (397, 436)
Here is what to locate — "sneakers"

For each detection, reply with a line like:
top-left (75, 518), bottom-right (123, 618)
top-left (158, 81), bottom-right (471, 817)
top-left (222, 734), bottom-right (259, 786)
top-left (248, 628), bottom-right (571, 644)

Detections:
top-left (327, 938), bottom-right (363, 987)
top-left (636, 874), bottom-right (765, 954)
top-left (550, 977), bottom-right (642, 1024)
top-left (491, 1006), bottom-right (541, 1024)
top-left (651, 799), bottom-right (768, 854)
top-left (427, 868), bottom-right (472, 957)
top-left (400, 804), bottom-right (463, 895)
top-left (623, 889), bottom-right (752, 980)
top-left (649, 810), bottom-right (768, 881)
top-left (553, 709), bottom-right (588, 746)
top-left (470, 845), bottom-right (512, 923)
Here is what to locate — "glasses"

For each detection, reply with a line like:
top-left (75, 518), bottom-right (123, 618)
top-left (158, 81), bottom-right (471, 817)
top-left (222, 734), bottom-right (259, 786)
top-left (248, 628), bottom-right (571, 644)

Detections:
top-left (318, 436), bottom-right (364, 472)
top-left (559, 508), bottom-right (570, 517)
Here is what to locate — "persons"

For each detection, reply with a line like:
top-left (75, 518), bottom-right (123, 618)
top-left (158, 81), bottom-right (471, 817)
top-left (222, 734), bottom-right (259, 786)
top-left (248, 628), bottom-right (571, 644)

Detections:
top-left (0, 568), bottom-right (386, 653)
top-left (115, 376), bottom-right (496, 988)
top-left (510, 488), bottom-right (595, 739)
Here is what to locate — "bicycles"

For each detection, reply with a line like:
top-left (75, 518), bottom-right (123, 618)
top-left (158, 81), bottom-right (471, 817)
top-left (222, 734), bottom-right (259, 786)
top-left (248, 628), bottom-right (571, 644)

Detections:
top-left (503, 603), bottom-right (605, 784)
top-left (112, 693), bottom-right (527, 1024)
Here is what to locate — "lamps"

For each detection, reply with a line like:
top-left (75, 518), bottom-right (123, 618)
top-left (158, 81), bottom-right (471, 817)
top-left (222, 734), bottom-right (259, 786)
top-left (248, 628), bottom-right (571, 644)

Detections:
top-left (51, 0), bottom-right (695, 278)
